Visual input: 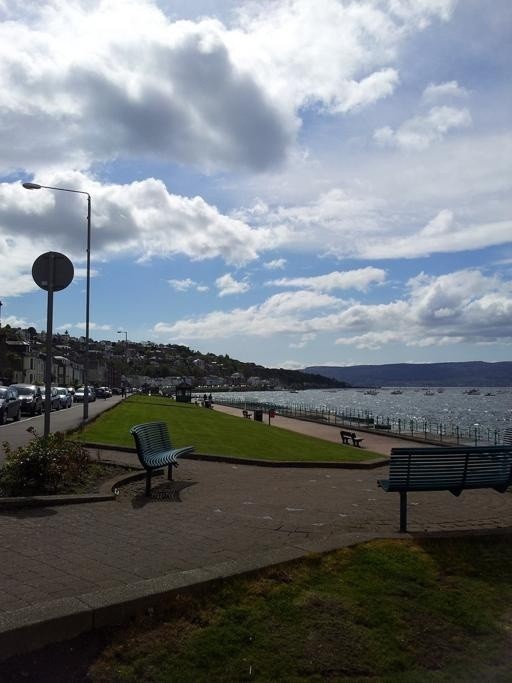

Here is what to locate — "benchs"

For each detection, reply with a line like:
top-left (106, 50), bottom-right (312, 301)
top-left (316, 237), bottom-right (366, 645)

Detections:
top-left (376, 445), bottom-right (512, 534)
top-left (130, 421), bottom-right (196, 498)
top-left (340, 431), bottom-right (363, 446)
top-left (243, 411), bottom-right (252, 419)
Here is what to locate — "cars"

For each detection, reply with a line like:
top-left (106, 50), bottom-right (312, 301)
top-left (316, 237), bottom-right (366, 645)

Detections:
top-left (0, 383), bottom-right (71, 424)
top-left (68, 385), bottom-right (137, 402)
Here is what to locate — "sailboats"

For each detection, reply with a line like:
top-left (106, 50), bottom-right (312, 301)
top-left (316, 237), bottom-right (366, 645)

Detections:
top-left (463, 383), bottom-right (512, 396)
top-left (366, 384), bottom-right (434, 396)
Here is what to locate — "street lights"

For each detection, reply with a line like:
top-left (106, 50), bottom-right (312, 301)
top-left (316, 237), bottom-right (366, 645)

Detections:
top-left (23, 183), bottom-right (91, 419)
top-left (117, 331), bottom-right (127, 398)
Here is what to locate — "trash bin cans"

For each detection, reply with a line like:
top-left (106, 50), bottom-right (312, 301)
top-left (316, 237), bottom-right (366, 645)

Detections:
top-left (254, 410), bottom-right (263, 422)
top-left (205, 400), bottom-right (209, 408)
